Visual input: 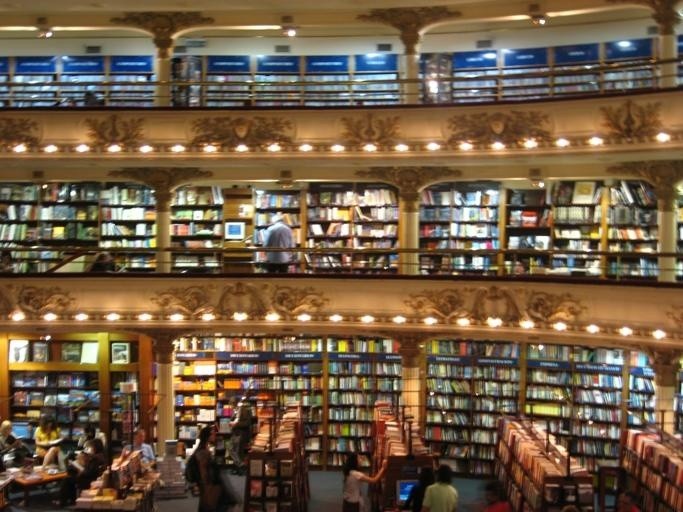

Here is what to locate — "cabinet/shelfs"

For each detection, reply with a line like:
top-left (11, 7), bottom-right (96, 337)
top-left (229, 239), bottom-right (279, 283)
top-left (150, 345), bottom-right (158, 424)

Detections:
top-left (0, 52), bottom-right (682, 511)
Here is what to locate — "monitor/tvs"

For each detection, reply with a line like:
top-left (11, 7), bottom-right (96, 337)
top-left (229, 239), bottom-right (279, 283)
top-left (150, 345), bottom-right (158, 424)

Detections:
top-left (224, 221), bottom-right (247, 241)
top-left (396, 480), bottom-right (418, 507)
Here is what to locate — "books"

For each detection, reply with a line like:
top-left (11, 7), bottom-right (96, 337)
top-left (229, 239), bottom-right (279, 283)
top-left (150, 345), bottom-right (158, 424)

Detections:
top-left (171, 74), bottom-right (403, 107)
top-left (1, 75), bottom-right (154, 108)
top-left (1, 182), bottom-right (157, 274)
top-left (418, 62), bottom-right (681, 108)
top-left (1, 330), bottom-right (683, 511)
top-left (418, 181), bottom-right (683, 282)
top-left (171, 183), bottom-right (399, 274)
